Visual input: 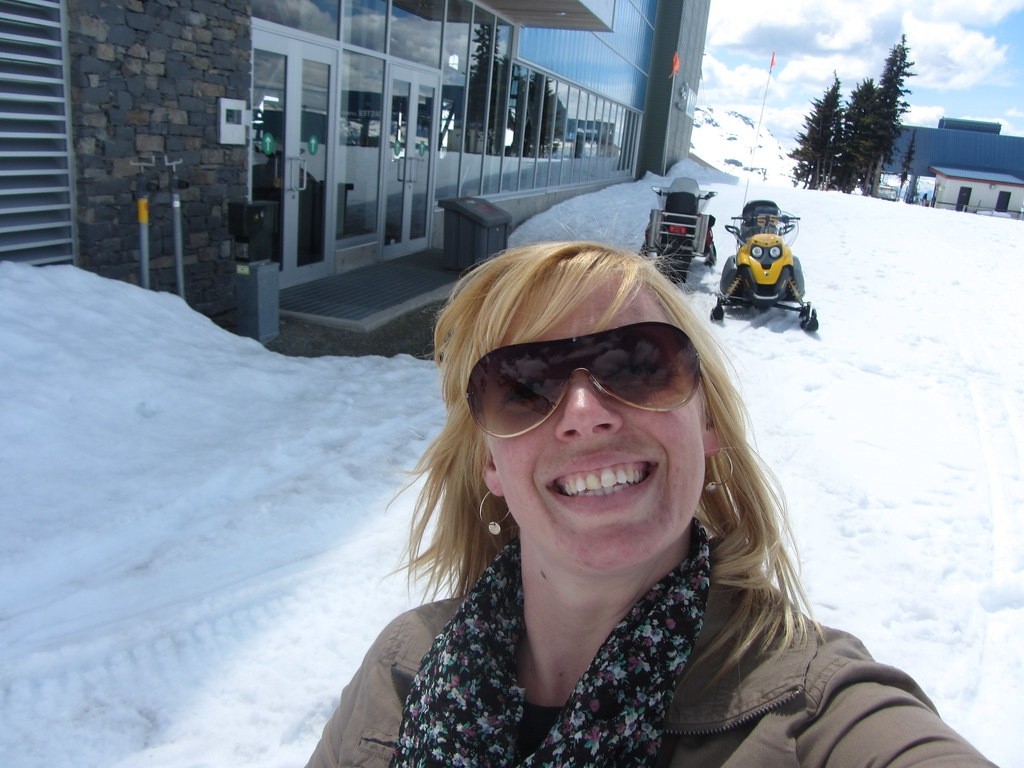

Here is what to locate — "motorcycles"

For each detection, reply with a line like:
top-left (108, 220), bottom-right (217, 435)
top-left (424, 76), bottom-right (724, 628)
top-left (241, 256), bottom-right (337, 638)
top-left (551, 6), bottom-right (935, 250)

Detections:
top-left (709, 200), bottom-right (820, 332)
top-left (637, 176), bottom-right (719, 287)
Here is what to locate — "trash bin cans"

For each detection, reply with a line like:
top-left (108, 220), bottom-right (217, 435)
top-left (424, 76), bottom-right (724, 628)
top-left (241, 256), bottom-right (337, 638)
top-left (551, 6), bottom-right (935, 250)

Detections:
top-left (437, 196), bottom-right (512, 272)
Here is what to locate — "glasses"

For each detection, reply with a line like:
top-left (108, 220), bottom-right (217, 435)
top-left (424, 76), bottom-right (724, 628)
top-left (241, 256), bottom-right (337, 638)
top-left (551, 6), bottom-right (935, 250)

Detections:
top-left (465, 320), bottom-right (703, 440)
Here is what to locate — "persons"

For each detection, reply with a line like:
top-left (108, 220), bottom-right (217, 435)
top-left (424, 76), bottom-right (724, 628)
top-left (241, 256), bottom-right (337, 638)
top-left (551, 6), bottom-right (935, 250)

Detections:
top-left (301, 241), bottom-right (994, 766)
